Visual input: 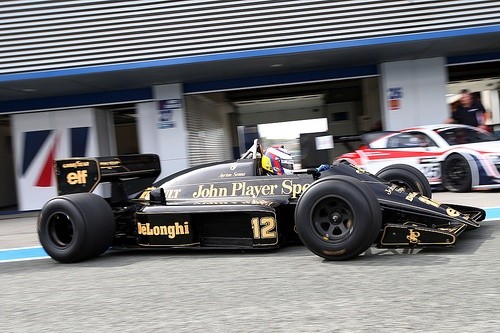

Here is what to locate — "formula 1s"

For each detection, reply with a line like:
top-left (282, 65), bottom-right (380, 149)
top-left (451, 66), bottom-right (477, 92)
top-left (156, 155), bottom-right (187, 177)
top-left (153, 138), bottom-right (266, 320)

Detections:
top-left (36, 137), bottom-right (486, 265)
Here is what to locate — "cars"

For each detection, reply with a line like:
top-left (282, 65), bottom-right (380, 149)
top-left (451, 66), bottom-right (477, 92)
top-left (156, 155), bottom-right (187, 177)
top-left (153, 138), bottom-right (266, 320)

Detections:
top-left (330, 122), bottom-right (500, 193)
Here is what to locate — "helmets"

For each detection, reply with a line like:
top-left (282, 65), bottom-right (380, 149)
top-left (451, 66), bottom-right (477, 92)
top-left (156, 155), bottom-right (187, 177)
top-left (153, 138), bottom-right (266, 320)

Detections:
top-left (262, 144), bottom-right (294, 175)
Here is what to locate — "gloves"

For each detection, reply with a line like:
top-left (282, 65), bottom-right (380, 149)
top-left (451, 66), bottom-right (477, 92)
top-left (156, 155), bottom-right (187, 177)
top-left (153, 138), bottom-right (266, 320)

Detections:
top-left (317, 165), bottom-right (329, 171)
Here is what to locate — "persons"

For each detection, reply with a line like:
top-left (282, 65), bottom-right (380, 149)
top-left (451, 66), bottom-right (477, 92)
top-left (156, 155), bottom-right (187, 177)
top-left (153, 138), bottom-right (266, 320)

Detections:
top-left (260, 144), bottom-right (329, 175)
top-left (444, 92), bottom-right (488, 141)
